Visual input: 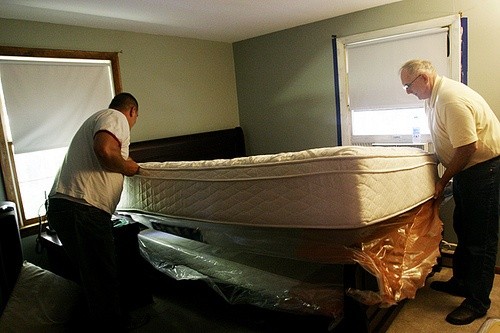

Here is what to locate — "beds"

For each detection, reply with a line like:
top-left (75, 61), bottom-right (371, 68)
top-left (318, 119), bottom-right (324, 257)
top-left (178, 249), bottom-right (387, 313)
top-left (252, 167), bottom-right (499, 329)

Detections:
top-left (125, 127), bottom-right (443, 333)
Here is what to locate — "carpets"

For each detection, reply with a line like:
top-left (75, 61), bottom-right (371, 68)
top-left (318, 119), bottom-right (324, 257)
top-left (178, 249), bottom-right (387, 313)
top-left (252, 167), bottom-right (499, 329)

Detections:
top-left (366, 265), bottom-right (500, 333)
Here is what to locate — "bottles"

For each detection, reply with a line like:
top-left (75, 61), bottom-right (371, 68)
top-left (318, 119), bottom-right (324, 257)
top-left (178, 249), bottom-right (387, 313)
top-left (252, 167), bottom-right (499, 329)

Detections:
top-left (412, 127), bottom-right (422, 145)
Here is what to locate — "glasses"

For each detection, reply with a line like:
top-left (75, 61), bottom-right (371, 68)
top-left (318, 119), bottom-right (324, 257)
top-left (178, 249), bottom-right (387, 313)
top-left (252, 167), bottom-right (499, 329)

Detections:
top-left (403, 75), bottom-right (421, 89)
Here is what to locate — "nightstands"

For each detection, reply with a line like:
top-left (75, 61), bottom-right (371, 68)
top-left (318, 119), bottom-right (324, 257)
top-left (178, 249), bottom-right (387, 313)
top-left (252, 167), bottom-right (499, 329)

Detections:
top-left (38, 216), bottom-right (139, 255)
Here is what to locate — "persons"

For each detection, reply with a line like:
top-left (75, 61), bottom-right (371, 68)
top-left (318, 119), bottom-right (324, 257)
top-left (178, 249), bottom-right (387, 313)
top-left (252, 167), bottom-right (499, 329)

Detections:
top-left (45, 92), bottom-right (151, 333)
top-left (398, 60), bottom-right (499, 325)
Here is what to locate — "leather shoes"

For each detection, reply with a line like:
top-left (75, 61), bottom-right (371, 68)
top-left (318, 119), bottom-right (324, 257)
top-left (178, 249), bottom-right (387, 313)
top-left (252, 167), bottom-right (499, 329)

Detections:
top-left (445, 298), bottom-right (487, 325)
top-left (430, 277), bottom-right (463, 296)
